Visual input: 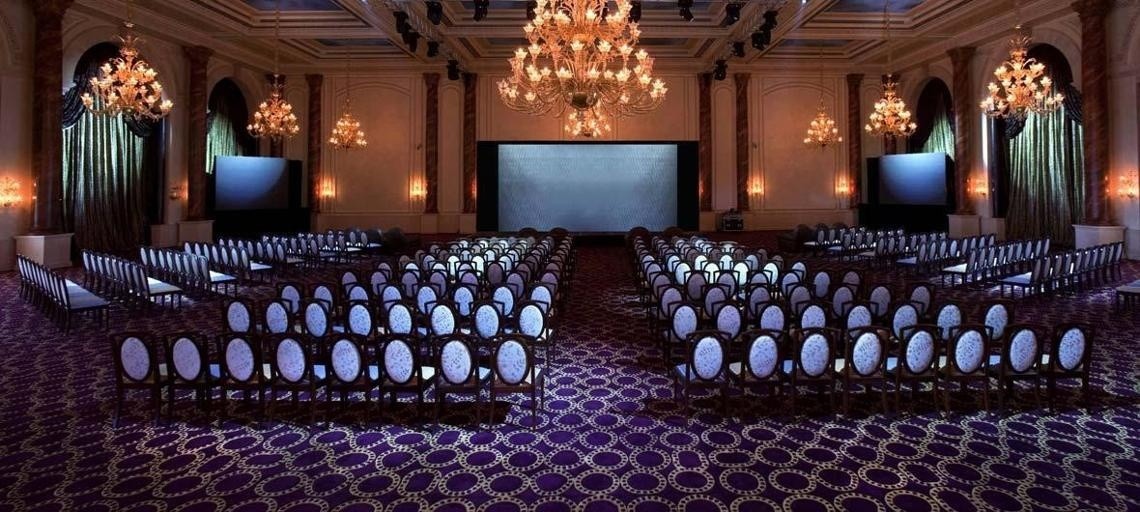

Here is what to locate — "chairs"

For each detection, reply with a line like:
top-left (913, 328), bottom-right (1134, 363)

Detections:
top-left (16, 227), bottom-right (578, 433)
top-left (628, 225), bottom-right (1140, 418)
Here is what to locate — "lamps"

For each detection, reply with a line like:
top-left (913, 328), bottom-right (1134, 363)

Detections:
top-left (4, 176), bottom-right (22, 203)
top-left (241, 2), bottom-right (300, 141)
top-left (862, 2), bottom-right (919, 140)
top-left (169, 184), bottom-right (182, 201)
top-left (979, 0), bottom-right (1065, 140)
top-left (407, 175), bottom-right (426, 207)
top-left (967, 172), bottom-right (990, 203)
top-left (746, 172), bottom-right (763, 204)
top-left (835, 172), bottom-right (857, 201)
top-left (1107, 173), bottom-right (1136, 202)
top-left (315, 174), bottom-right (339, 207)
top-left (803, 38), bottom-right (844, 153)
top-left (495, 2), bottom-right (671, 140)
top-left (327, 37), bottom-right (368, 151)
top-left (78, 2), bottom-right (177, 141)
